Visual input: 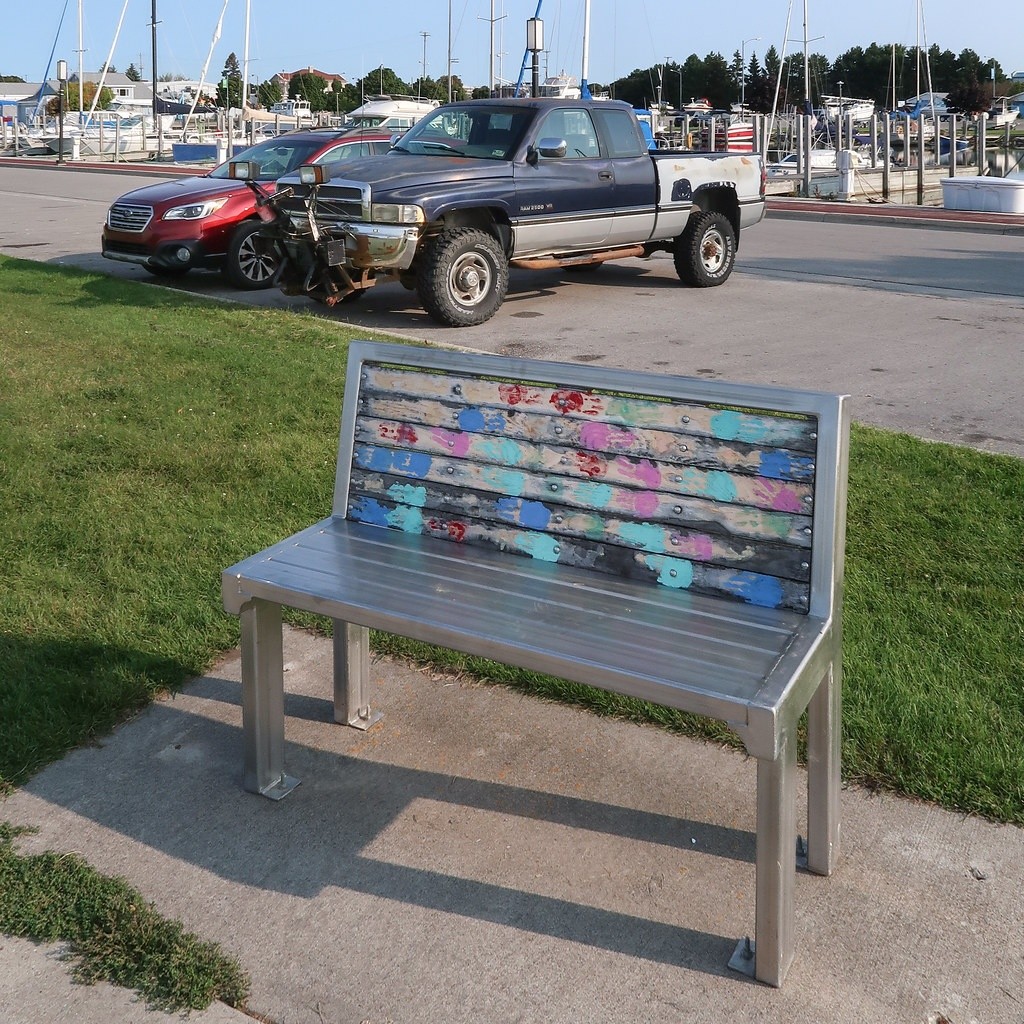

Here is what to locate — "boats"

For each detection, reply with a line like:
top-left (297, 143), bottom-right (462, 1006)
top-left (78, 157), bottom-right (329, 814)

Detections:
top-left (345, 95), bottom-right (444, 131)
top-left (700, 123), bottom-right (969, 177)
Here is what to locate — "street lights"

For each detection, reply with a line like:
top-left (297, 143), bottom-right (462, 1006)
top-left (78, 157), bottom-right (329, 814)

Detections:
top-left (670, 68), bottom-right (682, 111)
top-left (983, 56), bottom-right (996, 97)
top-left (742, 38), bottom-right (761, 102)
top-left (527, 17), bottom-right (544, 99)
top-left (56, 60), bottom-right (67, 163)
top-left (252, 75), bottom-right (260, 91)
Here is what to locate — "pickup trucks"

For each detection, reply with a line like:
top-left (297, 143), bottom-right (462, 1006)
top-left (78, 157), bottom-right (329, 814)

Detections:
top-left (276, 99), bottom-right (766, 328)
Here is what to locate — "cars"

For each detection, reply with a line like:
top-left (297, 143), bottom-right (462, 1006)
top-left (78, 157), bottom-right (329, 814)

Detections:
top-left (101, 128), bottom-right (467, 291)
top-left (906, 94), bottom-right (965, 122)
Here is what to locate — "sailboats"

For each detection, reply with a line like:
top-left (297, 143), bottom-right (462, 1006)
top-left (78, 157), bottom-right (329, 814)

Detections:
top-left (40, 0), bottom-right (353, 165)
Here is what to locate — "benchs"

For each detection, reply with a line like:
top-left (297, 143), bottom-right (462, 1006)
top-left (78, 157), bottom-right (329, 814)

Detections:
top-left (220, 341), bottom-right (850, 988)
